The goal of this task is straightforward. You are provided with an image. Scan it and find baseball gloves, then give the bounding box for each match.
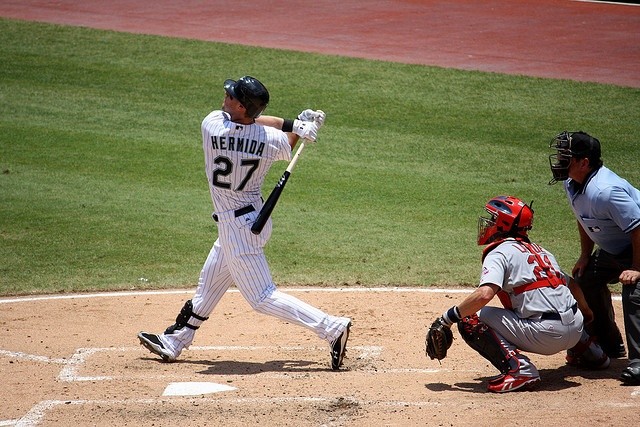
[425,318,454,361]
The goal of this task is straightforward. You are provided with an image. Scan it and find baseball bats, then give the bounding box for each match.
[250,110,326,236]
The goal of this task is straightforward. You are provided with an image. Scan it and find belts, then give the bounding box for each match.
[212,197,264,222]
[542,303,578,320]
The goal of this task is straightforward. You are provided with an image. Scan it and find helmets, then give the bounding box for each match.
[548,131,601,185]
[477,195,535,245]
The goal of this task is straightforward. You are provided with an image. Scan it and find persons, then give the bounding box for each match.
[543,132,640,386]
[138,75,352,371]
[425,194,612,393]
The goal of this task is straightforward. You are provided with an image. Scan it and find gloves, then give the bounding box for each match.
[292,119,317,142]
[298,108,326,126]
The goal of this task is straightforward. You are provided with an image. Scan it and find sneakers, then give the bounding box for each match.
[618,361,640,383]
[488,373,541,393]
[138,331,174,362]
[330,316,353,370]
[565,347,610,370]
[599,340,626,357]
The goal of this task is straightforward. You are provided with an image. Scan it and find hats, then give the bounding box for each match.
[223,76,269,118]
